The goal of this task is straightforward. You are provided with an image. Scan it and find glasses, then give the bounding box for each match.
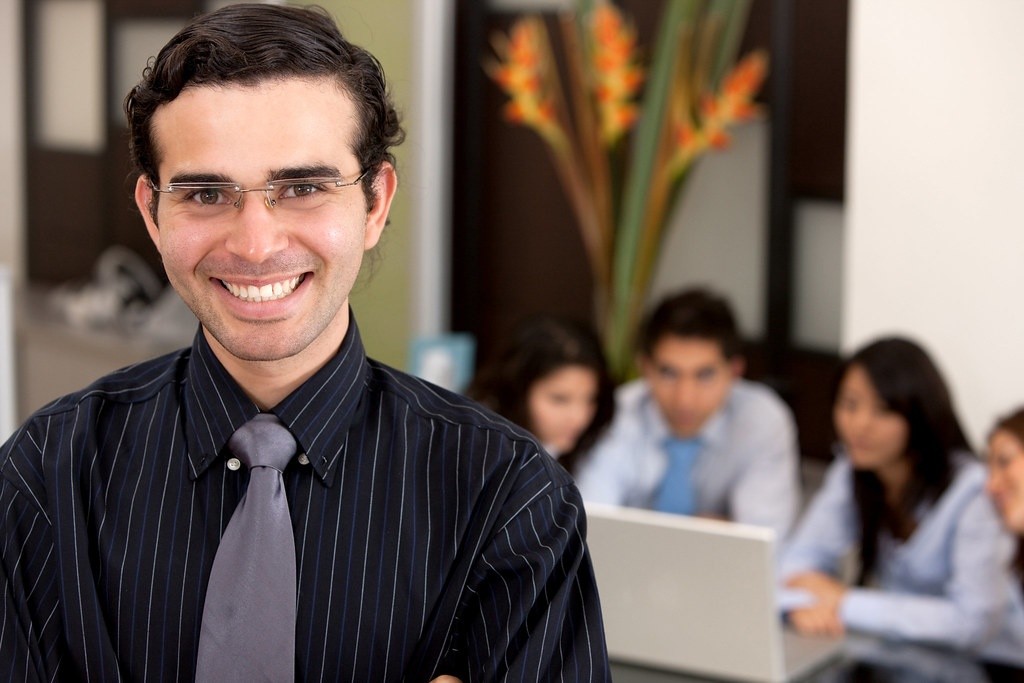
[146,167,373,223]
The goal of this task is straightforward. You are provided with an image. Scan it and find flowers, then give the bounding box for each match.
[483,0,778,390]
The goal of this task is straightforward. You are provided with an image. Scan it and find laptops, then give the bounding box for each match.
[580,504,849,683]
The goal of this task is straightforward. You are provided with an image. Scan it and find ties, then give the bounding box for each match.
[649,441,702,515]
[195,415,296,683]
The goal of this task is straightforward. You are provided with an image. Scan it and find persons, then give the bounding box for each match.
[571,290,797,556]
[0,5,611,683]
[782,341,1024,682]
[463,311,616,476]
